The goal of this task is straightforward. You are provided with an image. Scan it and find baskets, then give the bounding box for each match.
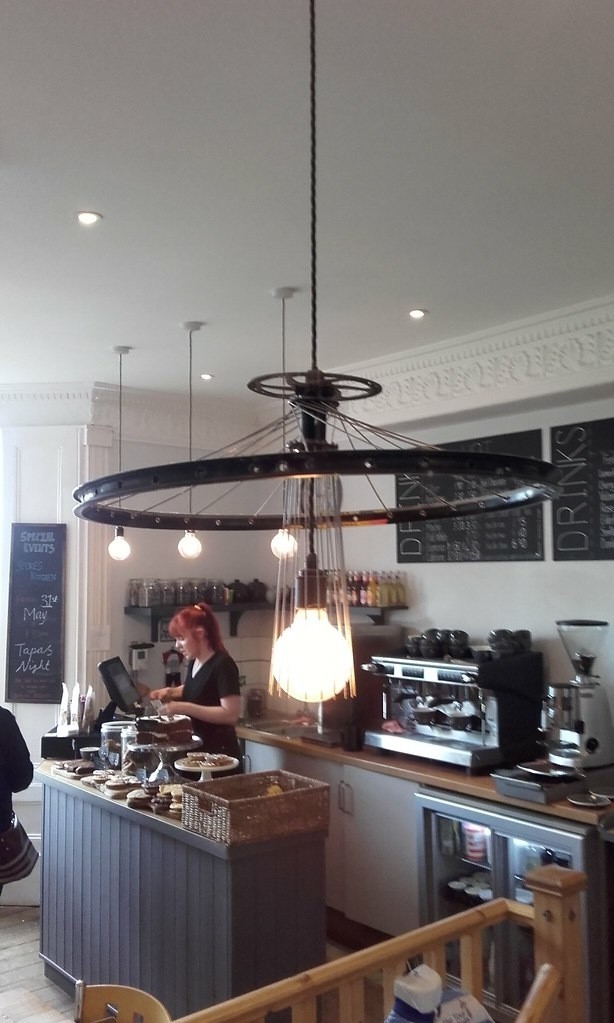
[181,769,331,844]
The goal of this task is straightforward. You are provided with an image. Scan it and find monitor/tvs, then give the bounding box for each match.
[98,656,141,712]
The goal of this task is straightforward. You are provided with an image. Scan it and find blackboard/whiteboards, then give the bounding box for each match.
[395,429,545,564]
[549,417,614,561]
[5,523,68,704]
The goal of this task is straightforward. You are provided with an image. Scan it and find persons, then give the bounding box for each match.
[148,602,244,781]
[0,707,33,896]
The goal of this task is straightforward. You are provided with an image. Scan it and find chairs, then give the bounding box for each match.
[75,979,171,1023]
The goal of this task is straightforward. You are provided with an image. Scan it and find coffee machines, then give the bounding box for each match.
[536,620,614,770]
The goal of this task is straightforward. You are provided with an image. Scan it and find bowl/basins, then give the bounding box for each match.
[80,747,100,761]
[448,870,535,905]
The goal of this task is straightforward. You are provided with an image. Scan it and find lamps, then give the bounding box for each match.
[71,1,567,705]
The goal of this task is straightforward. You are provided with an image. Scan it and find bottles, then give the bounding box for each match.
[326,569,405,608]
[128,577,290,605]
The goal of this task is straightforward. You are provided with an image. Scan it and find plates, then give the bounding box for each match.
[566,787,614,806]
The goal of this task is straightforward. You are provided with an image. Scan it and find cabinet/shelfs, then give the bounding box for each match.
[234,739,422,941]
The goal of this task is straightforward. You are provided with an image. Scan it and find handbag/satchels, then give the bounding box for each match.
[0,814,39,885]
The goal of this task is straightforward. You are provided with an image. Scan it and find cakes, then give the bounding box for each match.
[135,713,193,746]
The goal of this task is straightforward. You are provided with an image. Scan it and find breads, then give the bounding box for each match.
[63,761,190,812]
[186,752,228,766]
